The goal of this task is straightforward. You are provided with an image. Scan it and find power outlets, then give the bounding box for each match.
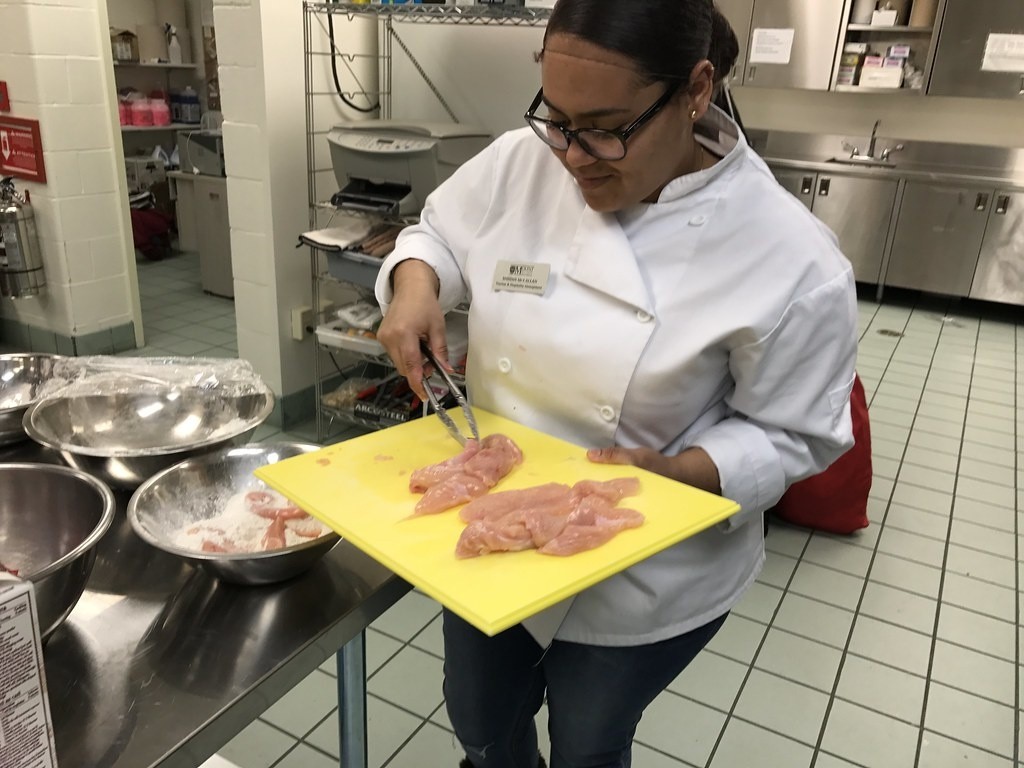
[312,299,334,335]
[292,305,316,341]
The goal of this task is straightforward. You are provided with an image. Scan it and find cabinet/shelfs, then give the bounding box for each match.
[193,180,234,299]
[112,59,208,254]
[767,166,901,301]
[301,0,556,443]
[876,181,1024,306]
[830,0,947,96]
[927,0,1024,100]
[713,0,846,91]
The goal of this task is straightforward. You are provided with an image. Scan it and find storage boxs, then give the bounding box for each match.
[175,130,225,176]
[123,155,166,193]
[109,25,146,61]
[0,567,59,768]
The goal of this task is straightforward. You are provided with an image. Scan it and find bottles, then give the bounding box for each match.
[151,145,170,171]
[117,91,171,126]
[170,145,179,171]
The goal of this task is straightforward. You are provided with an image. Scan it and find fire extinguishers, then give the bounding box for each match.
[0,177,46,297]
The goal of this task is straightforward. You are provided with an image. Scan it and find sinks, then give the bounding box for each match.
[825,156,897,169]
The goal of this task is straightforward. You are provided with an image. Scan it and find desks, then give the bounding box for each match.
[0,349,415,768]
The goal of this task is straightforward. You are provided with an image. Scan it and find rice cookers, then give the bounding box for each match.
[169,88,201,123]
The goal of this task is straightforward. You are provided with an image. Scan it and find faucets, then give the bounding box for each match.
[867,120,881,157]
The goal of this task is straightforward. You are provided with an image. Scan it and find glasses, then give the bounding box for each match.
[524,81,684,161]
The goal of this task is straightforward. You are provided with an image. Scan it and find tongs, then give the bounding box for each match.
[418,338,480,448]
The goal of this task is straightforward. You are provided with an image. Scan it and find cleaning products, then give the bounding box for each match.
[164,21,182,63]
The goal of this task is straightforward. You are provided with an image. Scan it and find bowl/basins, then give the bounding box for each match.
[129,443,335,590]
[0,462,117,640]
[0,353,87,458]
[22,356,276,496]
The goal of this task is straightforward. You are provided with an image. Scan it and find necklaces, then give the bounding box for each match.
[697,144,706,172]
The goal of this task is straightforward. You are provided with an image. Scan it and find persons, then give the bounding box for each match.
[374,0,858,768]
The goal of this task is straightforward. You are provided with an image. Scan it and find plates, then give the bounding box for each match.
[255,406,743,637]
